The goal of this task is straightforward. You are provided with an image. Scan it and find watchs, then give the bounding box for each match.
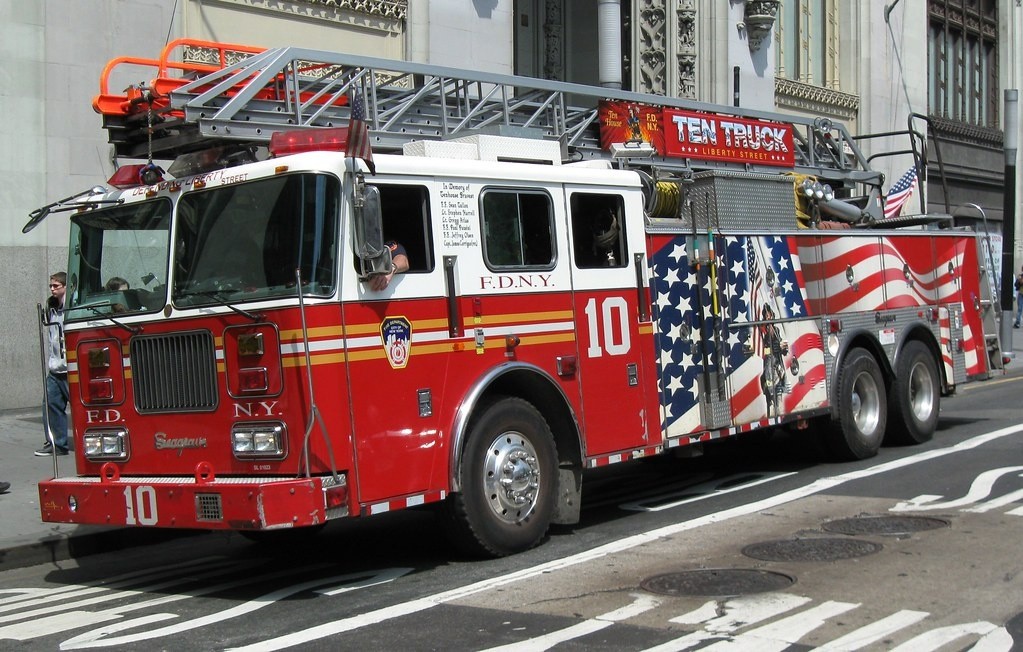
[391,263,398,273]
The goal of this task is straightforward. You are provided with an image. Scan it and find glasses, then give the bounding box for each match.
[70,285,74,289]
[49,284,63,288]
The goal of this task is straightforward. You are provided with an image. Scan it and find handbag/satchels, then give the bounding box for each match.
[1015,279,1021,290]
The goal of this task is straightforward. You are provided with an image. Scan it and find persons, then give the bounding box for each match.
[368,237,409,292]
[1011,265,1023,329]
[105,278,129,292]
[761,303,792,394]
[33,272,77,457]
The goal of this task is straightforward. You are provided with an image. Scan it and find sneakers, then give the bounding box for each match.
[34,441,68,455]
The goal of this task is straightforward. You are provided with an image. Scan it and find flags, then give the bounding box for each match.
[883,159,921,219]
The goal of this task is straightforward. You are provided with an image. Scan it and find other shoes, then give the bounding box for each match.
[1014,321,1020,328]
[0,481,11,493]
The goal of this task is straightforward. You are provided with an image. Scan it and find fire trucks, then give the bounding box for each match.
[22,38,1011,561]
